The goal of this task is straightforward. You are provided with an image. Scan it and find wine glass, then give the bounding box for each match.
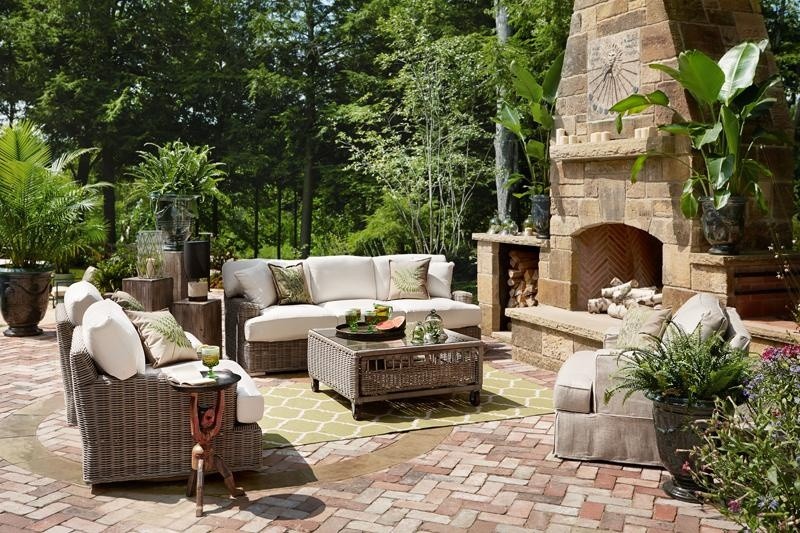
[345,308,378,334]
[202,346,220,380]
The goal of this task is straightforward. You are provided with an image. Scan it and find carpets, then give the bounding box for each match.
[251,365,555,449]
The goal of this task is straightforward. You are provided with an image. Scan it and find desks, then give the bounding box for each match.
[122,252,189,316]
[167,370,245,517]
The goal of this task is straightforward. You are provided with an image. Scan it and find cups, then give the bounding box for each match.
[524,228,532,236]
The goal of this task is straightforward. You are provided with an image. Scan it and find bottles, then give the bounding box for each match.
[411,309,443,344]
[487,214,534,236]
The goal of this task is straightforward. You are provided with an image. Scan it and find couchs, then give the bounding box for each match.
[54,281,264,484]
[222,254,482,377]
[553,293,752,467]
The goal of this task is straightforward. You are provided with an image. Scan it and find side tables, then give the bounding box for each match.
[173,298,222,359]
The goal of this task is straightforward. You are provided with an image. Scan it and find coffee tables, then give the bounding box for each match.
[308,322,485,420]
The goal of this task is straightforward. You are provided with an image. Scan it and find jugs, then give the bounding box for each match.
[374,304,393,325]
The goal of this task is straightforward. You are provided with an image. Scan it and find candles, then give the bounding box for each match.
[146,258,156,278]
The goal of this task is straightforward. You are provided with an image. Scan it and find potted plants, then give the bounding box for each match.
[123,137,233,251]
[608,37,796,256]
[604,317,763,506]
[489,49,566,239]
[0,117,116,337]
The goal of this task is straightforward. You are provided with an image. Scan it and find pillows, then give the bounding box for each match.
[124,307,201,369]
[388,257,432,300]
[617,302,671,353]
[426,261,455,298]
[234,261,277,310]
[112,291,144,311]
[266,262,315,305]
